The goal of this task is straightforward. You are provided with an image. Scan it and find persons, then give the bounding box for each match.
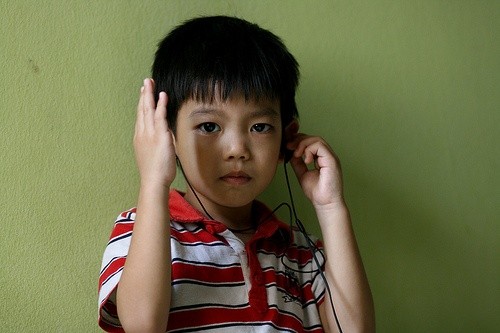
[97,16,376,333]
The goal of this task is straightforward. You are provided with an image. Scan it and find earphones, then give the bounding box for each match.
[281,139,295,163]
